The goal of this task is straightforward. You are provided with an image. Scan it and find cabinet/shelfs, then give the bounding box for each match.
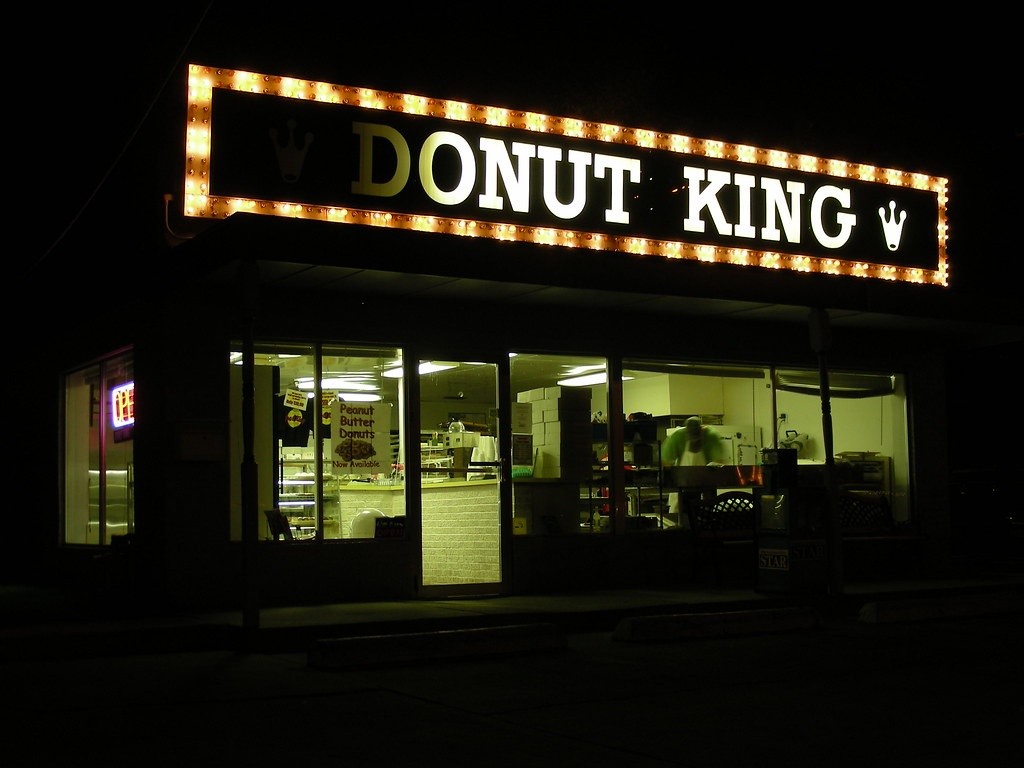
[279,447,341,539]
[388,417,496,480]
[585,411,665,533]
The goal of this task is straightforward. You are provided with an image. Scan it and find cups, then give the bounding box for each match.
[467,435,498,480]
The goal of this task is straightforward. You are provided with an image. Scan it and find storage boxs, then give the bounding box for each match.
[495,385,594,536]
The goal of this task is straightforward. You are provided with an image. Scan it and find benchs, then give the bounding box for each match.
[684,489,929,590]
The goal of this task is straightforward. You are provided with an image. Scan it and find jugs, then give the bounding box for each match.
[449,417,465,433]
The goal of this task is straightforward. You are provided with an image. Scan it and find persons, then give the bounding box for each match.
[657,415,728,514]
